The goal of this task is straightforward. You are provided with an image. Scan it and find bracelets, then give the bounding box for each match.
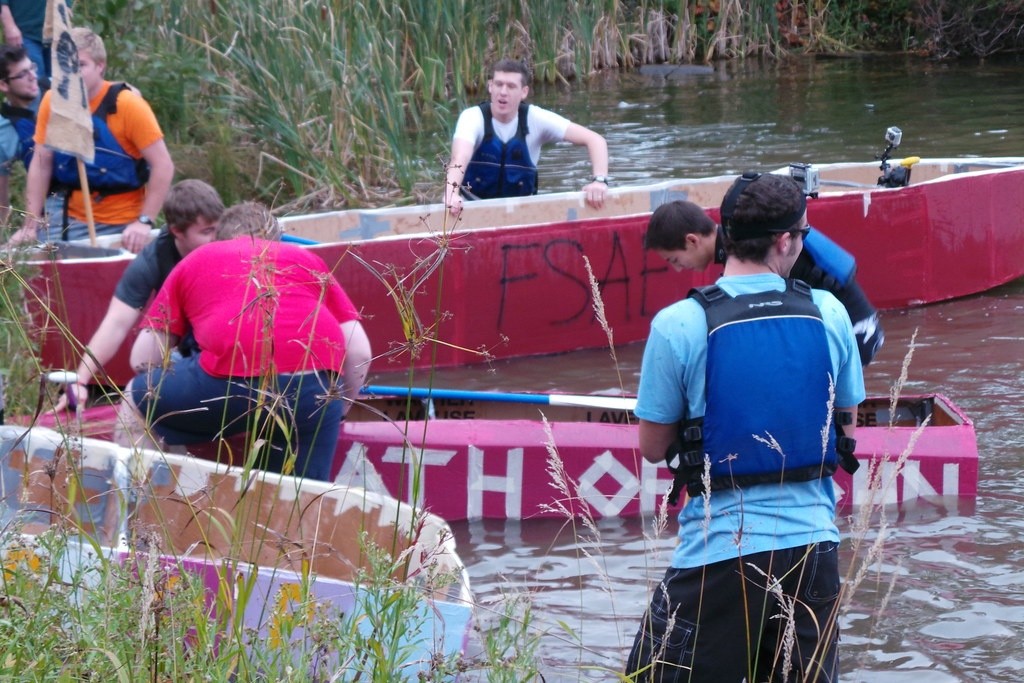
[341,415,347,421]
[76,381,87,387]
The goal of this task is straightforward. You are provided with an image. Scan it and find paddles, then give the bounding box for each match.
[360,384,637,411]
[280,234,320,245]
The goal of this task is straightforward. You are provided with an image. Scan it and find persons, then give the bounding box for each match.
[0,0,372,483]
[645,201,885,369]
[625,172,867,683]
[443,57,608,216]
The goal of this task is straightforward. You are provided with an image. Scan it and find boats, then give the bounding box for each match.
[1,392,977,522]
[0,156,1023,388]
[1,425,478,683]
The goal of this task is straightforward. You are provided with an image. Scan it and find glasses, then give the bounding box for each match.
[768,224,810,240]
[4,62,37,80]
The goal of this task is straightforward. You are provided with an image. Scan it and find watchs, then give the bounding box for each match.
[137,215,156,229]
[592,175,607,186]
